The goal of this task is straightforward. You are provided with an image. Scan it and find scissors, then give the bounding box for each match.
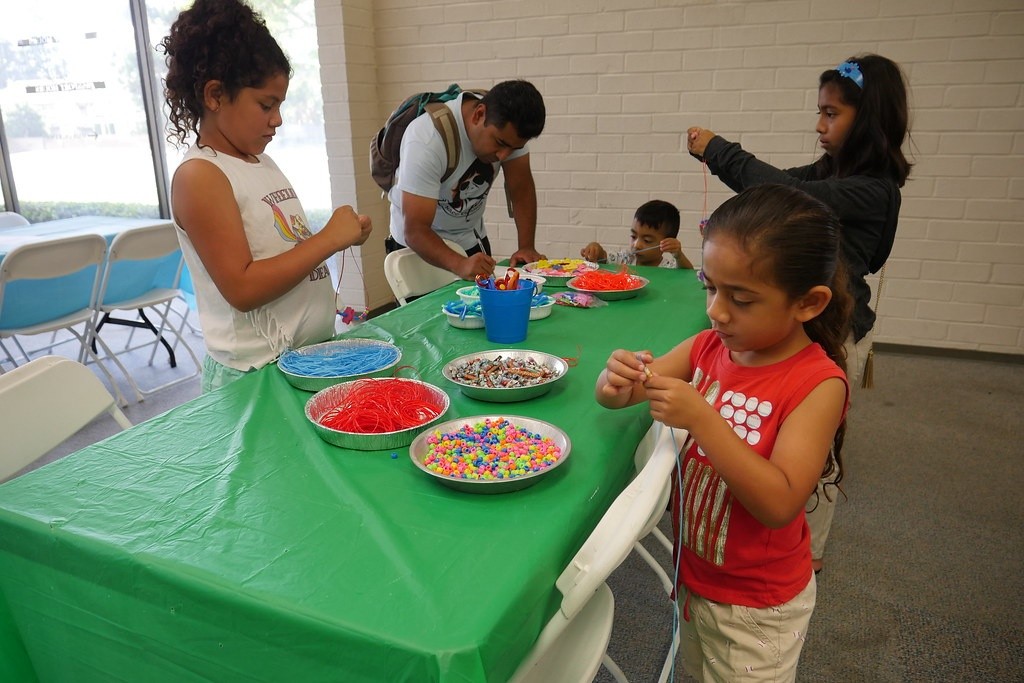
[507,272,519,290]
[476,274,489,287]
[494,268,516,290]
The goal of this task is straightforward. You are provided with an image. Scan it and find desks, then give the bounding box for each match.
[0,214,179,369]
[0,252,715,683]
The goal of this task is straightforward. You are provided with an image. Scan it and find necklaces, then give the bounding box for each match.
[697,152,710,283]
[334,243,374,325]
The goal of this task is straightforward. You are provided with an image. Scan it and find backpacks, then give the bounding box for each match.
[369,84,490,192]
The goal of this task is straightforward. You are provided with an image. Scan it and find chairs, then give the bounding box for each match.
[83,222,202,404]
[0,233,128,411]
[384,237,470,309]
[508,415,691,683]
[0,211,30,232]
[0,354,136,490]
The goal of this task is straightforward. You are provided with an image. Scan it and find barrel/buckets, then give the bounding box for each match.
[475,278,538,344]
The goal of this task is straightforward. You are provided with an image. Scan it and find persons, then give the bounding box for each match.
[156,0,374,391]
[580,199,694,269]
[369,79,553,309]
[594,184,851,683]
[687,52,916,574]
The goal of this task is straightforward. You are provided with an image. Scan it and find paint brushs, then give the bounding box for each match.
[473,228,497,279]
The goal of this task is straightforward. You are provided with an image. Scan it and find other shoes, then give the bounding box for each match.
[808,559,822,575]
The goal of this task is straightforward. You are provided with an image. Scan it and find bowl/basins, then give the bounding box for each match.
[442,308,485,329]
[522,260,600,287]
[528,294,557,321]
[303,377,451,451]
[408,414,572,494]
[456,286,481,305]
[566,273,650,301]
[443,348,569,403]
[277,338,403,393]
[518,275,546,294]
